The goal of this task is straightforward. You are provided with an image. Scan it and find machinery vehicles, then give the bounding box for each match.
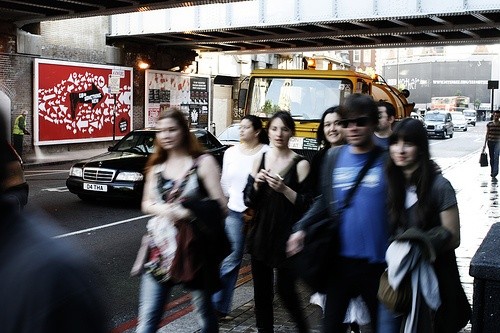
[231,57,416,154]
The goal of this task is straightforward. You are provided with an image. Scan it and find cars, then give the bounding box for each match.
[452,111,467,131]
[218,122,245,148]
[424,111,453,139]
[66,129,228,201]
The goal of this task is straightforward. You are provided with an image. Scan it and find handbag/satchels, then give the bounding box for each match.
[130,235,152,276]
[294,215,342,295]
[479,148,488,167]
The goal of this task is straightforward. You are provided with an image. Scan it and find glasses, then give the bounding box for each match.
[338,117,372,128]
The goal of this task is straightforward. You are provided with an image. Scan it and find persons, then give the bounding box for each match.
[220,92,471,333]
[129,107,234,333]
[12,110,30,160]
[479,110,500,182]
[1,90,113,333]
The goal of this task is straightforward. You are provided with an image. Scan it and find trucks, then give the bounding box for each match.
[462,107,476,127]
